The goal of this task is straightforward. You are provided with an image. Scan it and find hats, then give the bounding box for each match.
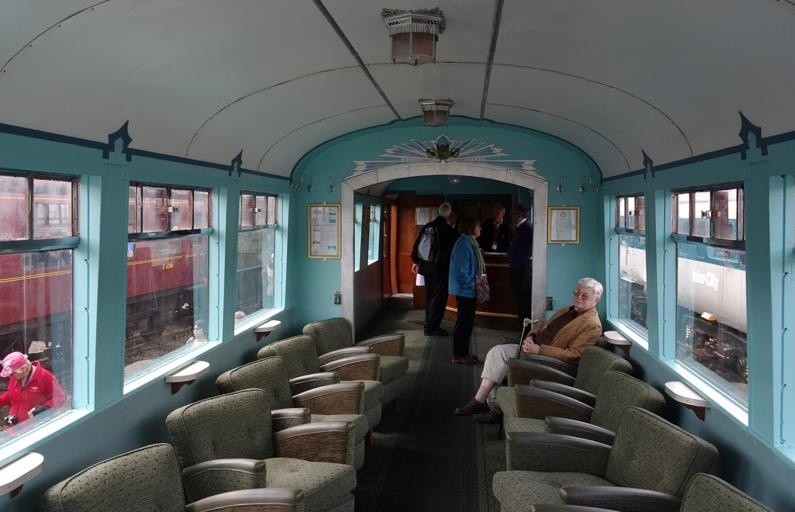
[0,352,29,378]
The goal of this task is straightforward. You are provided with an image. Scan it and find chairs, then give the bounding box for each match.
[302,317,410,412]
[216,355,369,470]
[486,334,606,412]
[530,472,771,512]
[41,443,304,512]
[496,344,633,416]
[492,406,720,512]
[166,388,357,512]
[503,371,666,445]
[256,335,383,443]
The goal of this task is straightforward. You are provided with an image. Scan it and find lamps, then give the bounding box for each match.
[449,176,462,184]
[417,97,453,127]
[382,7,445,66]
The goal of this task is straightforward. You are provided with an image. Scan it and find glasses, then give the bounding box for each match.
[573,288,589,297]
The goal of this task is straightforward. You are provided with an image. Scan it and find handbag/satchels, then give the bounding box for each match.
[476,273,491,304]
[417,233,435,262]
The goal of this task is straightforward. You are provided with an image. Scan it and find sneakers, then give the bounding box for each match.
[455,397,490,415]
[475,408,503,422]
[453,354,478,365]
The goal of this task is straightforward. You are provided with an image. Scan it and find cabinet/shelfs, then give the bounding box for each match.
[448,193,512,235]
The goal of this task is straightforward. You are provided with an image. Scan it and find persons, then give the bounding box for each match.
[0,351,67,432]
[448,215,488,365]
[235,311,246,320]
[454,278,603,425]
[473,201,516,257]
[186,319,208,343]
[507,202,533,333]
[411,202,459,336]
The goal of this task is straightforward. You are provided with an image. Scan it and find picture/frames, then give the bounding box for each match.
[308,203,342,259]
[548,206,580,244]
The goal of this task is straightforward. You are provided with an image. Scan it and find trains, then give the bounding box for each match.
[617,189,747,364]
[1,176,269,390]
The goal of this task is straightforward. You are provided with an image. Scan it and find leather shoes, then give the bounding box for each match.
[424,328,449,336]
